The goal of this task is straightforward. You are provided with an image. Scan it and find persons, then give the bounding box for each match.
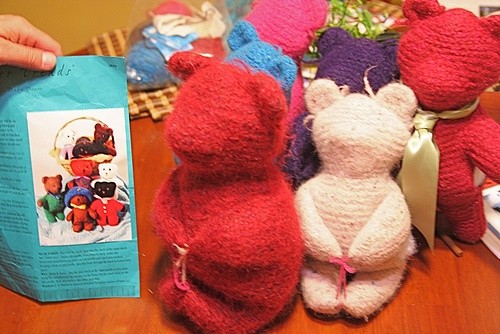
[0,14,64,74]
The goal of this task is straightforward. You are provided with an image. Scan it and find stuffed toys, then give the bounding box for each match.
[64,185,96,232]
[59,127,76,159]
[148,51,303,333]
[240,0,330,59]
[126,40,169,91]
[89,182,127,225]
[295,77,418,320]
[284,27,397,191]
[218,20,298,91]
[92,123,117,156]
[72,137,92,158]
[37,174,64,223]
[64,160,95,188]
[90,163,125,190]
[397,1,500,243]
